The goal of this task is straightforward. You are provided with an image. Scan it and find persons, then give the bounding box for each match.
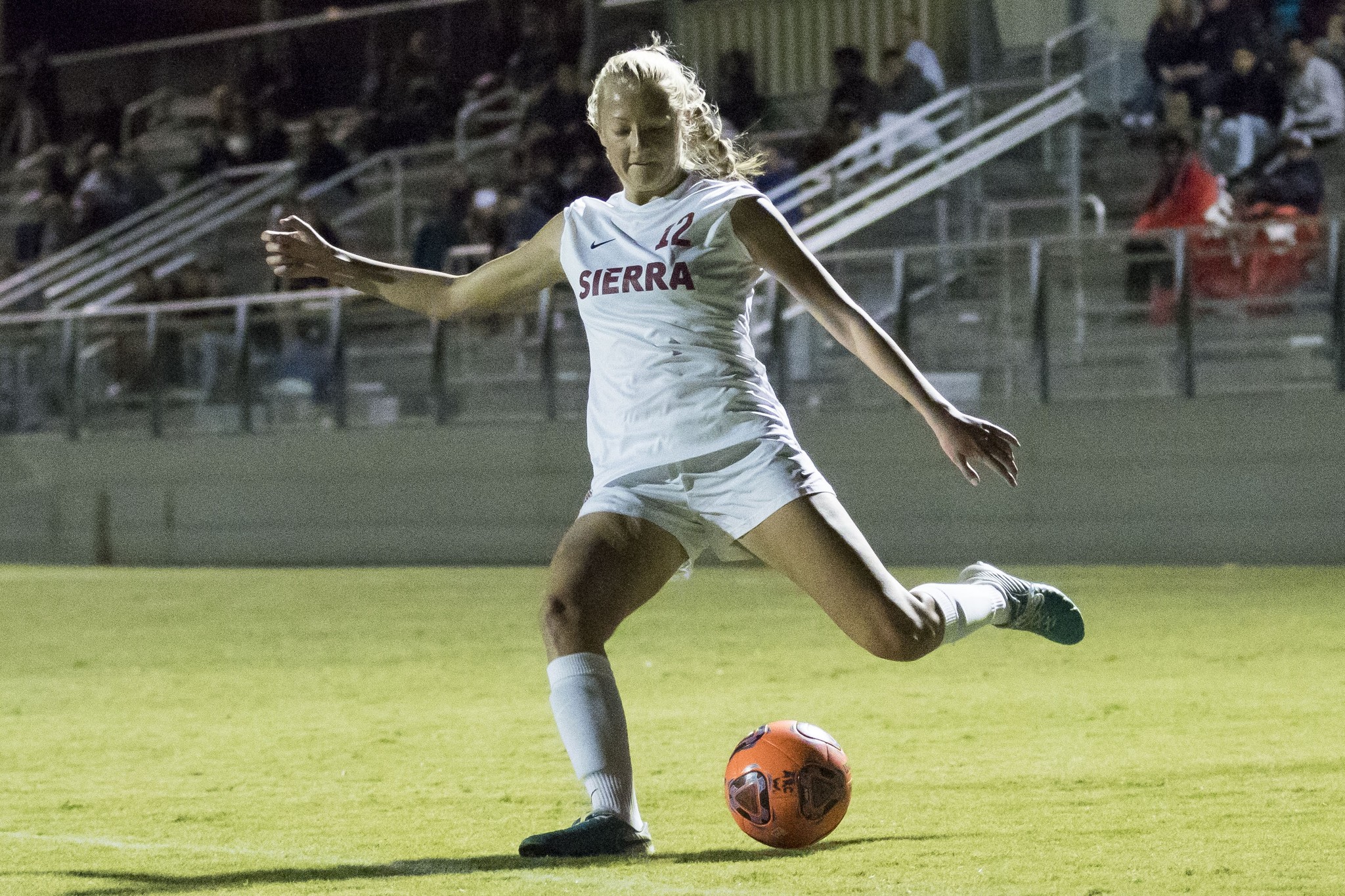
[0,1,952,400]
[1112,0,1340,319]
[256,46,1089,860]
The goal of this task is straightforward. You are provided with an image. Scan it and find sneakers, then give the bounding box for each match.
[959,560,1086,646]
[518,806,655,858]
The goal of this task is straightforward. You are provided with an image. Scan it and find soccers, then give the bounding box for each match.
[727,720,857,850]
